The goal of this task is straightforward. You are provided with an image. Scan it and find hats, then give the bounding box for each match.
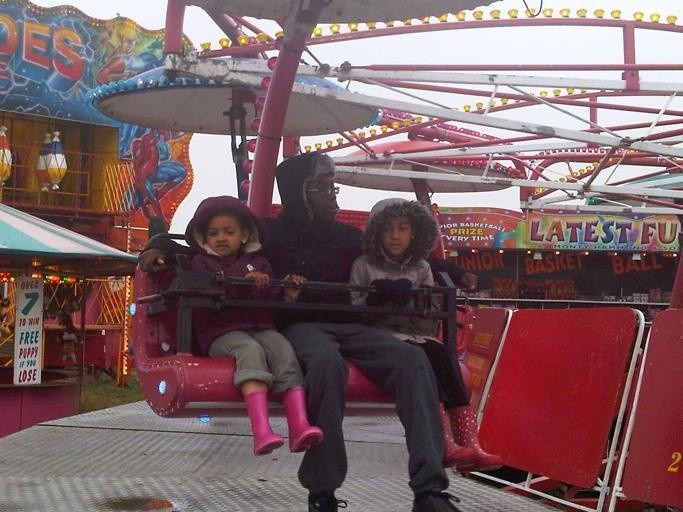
[272,152,335,221]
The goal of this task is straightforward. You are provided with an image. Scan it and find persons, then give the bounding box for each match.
[136,150,479,512]
[187,195,325,455]
[348,199,505,475]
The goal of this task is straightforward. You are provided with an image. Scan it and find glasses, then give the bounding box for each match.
[307,186,340,196]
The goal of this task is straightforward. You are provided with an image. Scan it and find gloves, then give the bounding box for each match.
[365,275,416,309]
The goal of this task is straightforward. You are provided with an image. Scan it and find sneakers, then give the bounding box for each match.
[411,490,463,512]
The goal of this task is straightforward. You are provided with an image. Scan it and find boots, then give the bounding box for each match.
[439,403,475,469]
[449,403,505,475]
[245,390,284,457]
[282,384,325,453]
